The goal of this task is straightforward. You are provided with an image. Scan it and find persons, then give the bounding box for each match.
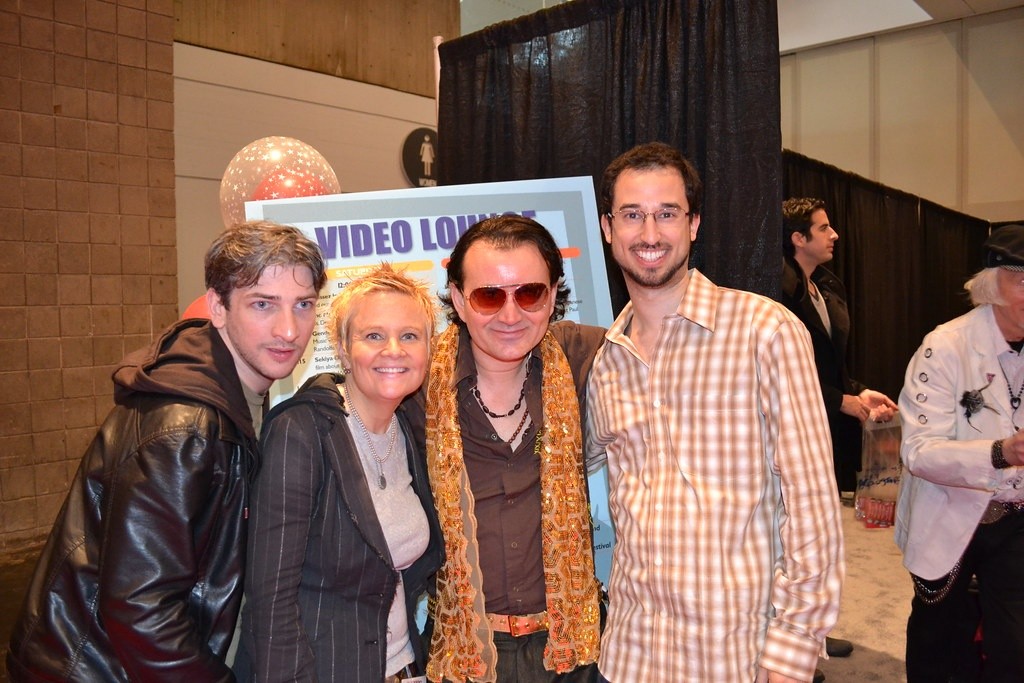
[583,144,845,683]
[6,222,328,683]
[415,215,609,683]
[228,261,447,682]
[895,226,1024,683]
[781,198,899,492]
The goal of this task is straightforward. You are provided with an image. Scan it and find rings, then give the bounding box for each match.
[882,419,885,423]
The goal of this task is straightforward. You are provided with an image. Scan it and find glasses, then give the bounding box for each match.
[605,207,693,228]
[454,281,553,316]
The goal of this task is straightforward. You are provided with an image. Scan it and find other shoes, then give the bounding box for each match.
[825,635,854,657]
[813,668,826,682]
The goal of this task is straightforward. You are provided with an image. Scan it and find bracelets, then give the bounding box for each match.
[991,440,1013,469]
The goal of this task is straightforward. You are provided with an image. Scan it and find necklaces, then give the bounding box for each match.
[997,356,1024,409]
[344,383,395,489]
[473,353,529,445]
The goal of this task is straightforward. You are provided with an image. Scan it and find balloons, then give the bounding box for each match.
[220,136,341,230]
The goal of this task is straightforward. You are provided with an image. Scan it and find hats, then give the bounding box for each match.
[982,224,1024,271]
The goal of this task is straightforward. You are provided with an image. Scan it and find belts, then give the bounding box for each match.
[474,607,550,639]
